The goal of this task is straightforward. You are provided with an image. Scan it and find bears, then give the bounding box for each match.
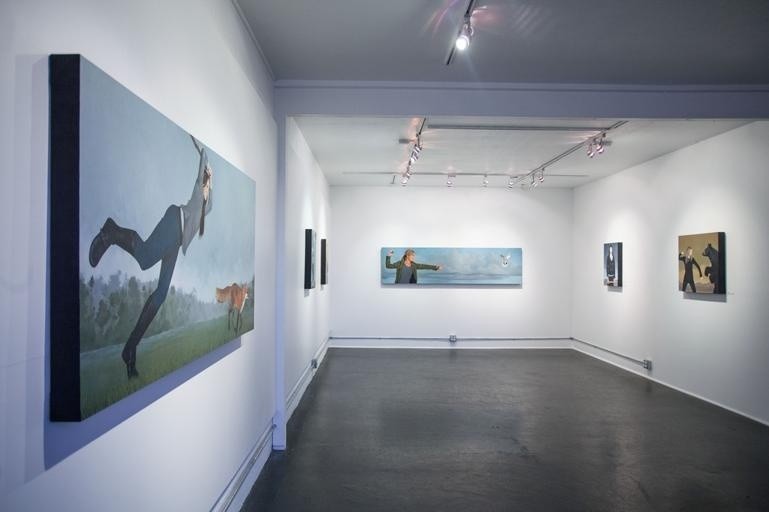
[702,243,720,293]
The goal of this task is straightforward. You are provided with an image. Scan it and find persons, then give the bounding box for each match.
[89,148,215,376]
[386,250,442,284]
[679,247,703,293]
[606,246,617,286]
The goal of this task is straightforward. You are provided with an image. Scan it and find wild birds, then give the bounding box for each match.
[500,254,511,265]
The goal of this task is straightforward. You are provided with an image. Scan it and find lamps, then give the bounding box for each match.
[586,136,605,160]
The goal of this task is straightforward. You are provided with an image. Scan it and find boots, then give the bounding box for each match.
[89,218,137,268]
[122,295,158,379]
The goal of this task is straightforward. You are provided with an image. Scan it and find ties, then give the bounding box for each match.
[199,198,207,235]
